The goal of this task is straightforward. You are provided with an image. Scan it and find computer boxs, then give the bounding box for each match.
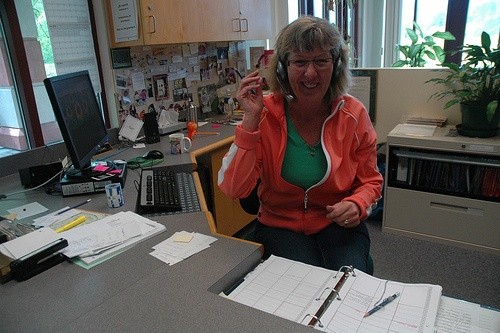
[61,159,127,196]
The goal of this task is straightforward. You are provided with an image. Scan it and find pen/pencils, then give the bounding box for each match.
[233,66,268,113]
[362,291,400,319]
[54,198,91,218]
[195,130,221,135]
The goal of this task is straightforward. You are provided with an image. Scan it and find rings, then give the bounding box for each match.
[344,218,349,225]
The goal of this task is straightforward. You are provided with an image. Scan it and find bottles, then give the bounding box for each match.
[224,88,232,112]
[186,105,198,127]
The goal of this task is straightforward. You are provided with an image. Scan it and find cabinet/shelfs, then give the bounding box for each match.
[382,124,500,255]
[102,0,247,48]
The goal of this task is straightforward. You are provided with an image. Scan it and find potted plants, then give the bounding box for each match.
[424,31,500,138]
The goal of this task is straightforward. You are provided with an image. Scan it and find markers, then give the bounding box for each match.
[47,216,86,232]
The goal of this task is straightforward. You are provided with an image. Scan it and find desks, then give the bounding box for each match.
[0,118,500,333]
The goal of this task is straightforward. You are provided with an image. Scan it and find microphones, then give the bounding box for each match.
[277,77,294,101]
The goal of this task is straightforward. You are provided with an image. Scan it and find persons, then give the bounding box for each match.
[218,15,384,275]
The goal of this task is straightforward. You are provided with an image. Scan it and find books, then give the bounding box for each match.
[408,156,500,199]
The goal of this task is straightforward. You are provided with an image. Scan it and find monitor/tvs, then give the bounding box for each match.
[43,69,115,179]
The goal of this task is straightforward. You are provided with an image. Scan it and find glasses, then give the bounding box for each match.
[283,54,335,71]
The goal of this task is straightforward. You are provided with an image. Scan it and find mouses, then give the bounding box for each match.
[142,150,164,160]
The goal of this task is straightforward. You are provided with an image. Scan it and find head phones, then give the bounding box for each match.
[276,45,343,81]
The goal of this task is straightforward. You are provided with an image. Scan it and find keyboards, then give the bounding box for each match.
[140,170,183,211]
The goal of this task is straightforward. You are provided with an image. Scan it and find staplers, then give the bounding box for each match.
[9,237,68,282]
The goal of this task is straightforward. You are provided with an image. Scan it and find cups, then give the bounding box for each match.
[187,122,197,136]
[169,133,191,155]
